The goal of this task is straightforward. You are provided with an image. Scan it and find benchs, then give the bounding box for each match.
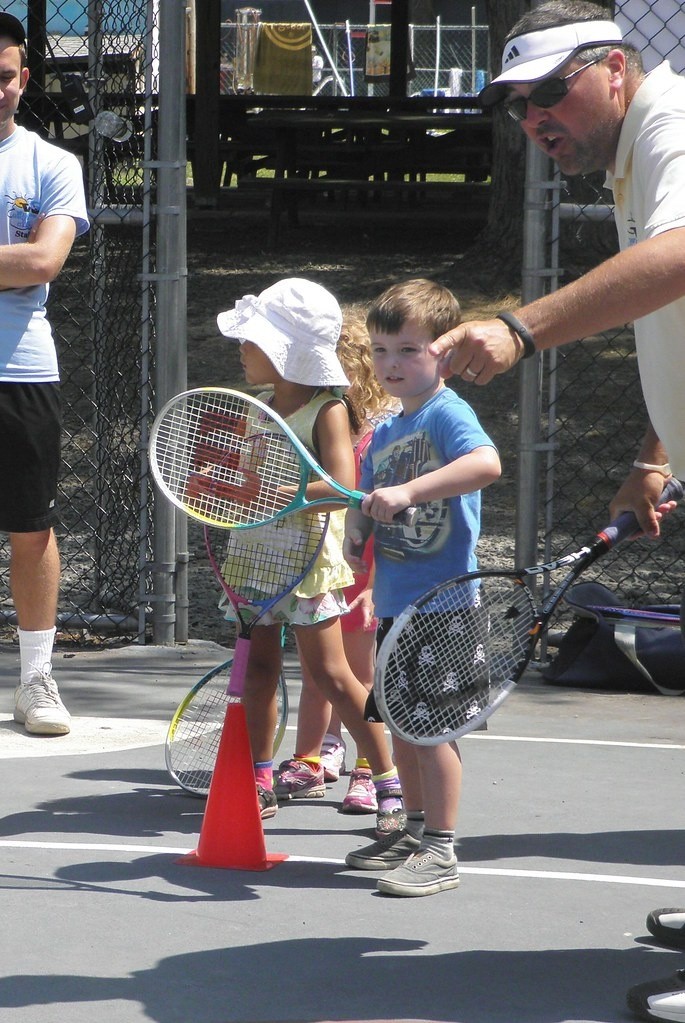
[89,94,487,232]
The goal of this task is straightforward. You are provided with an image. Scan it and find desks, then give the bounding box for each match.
[250,104,489,220]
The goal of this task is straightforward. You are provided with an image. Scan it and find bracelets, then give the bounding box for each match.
[632,459,672,476]
[495,312,535,359]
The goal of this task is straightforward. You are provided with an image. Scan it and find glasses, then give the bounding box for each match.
[222,56,228,59]
[503,55,609,122]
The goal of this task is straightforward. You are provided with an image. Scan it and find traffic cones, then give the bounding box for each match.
[170,703,290,874]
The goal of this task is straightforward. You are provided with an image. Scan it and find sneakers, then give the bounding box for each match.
[646,908,685,949]
[345,829,421,870]
[374,788,407,839]
[272,759,326,800]
[343,767,378,813]
[321,733,346,781]
[377,849,460,896]
[256,782,278,818]
[13,661,71,734]
[627,973,685,1023]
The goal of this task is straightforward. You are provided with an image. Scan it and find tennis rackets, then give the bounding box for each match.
[148,387,420,530]
[205,511,331,696]
[373,478,685,747]
[165,622,289,799]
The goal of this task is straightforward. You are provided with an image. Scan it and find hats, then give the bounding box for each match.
[0,12,27,54]
[217,278,351,398]
[477,19,624,106]
[311,46,317,51]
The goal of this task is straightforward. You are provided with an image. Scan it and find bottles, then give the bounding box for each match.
[95,111,132,142]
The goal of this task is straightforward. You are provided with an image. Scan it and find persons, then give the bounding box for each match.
[271,300,383,812]
[217,277,407,838]
[321,705,347,781]
[0,11,90,732]
[427,0,685,1023]
[342,277,500,896]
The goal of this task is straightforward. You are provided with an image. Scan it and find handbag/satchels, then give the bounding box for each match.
[543,581,685,696]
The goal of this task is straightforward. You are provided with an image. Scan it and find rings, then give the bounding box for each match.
[466,366,478,377]
